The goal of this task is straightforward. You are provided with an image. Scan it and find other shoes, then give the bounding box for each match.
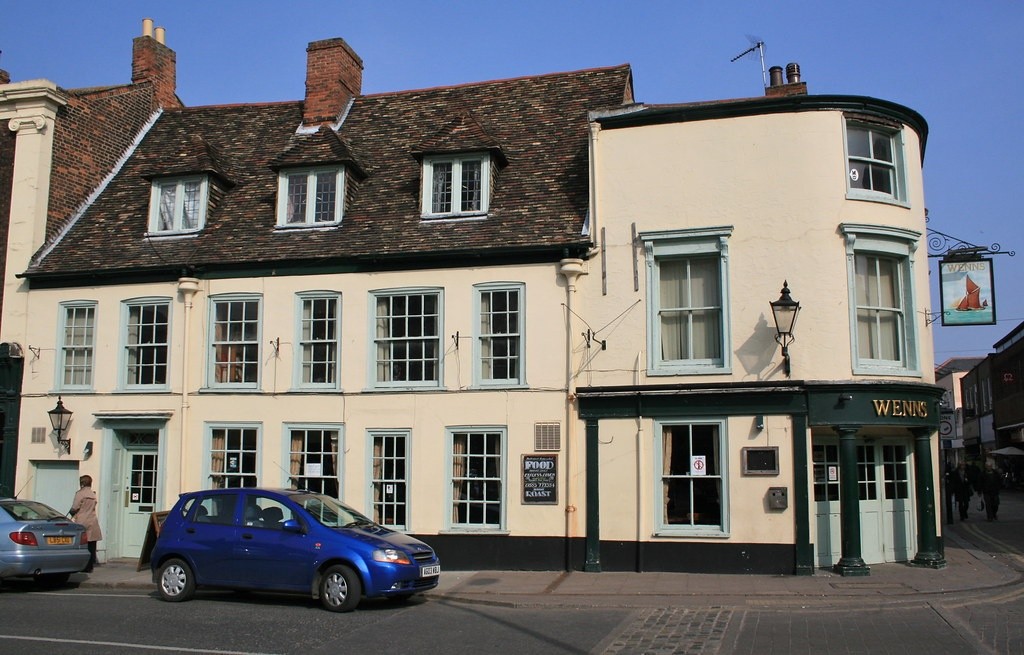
[81,567,93,572]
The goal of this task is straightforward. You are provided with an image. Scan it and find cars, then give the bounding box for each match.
[0,497,92,588]
[149,488,441,612]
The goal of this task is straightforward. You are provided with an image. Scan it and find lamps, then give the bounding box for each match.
[769,279,802,376]
[47,395,73,456]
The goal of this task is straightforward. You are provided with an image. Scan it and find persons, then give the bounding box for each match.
[946,459,1003,522]
[69,475,102,573]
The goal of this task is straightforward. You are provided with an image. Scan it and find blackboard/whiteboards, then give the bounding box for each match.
[137,511,171,572]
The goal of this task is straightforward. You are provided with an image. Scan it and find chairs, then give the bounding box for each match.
[247,505,283,530]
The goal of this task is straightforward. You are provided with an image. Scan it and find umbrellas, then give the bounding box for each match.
[988,446,1024,463]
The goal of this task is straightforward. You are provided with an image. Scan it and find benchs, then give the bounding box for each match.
[196,506,213,522]
